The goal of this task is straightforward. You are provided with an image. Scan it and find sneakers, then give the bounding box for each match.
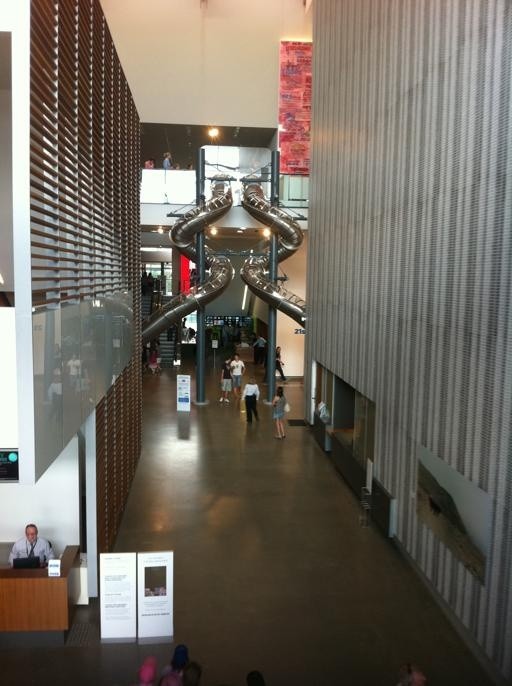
[220,397,229,402]
[274,433,286,439]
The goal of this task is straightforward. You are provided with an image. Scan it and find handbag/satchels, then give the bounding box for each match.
[283,403,290,413]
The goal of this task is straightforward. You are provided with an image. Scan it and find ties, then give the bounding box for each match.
[28,542,35,556]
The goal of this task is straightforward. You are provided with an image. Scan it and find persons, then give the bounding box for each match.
[272,386,287,439]
[158,644,189,686]
[8,525,54,567]
[47,333,120,423]
[246,671,265,686]
[397,662,425,685]
[139,656,157,686]
[144,151,195,171]
[142,269,290,426]
[183,661,201,685]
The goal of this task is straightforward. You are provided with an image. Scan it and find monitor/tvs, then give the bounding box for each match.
[13,556,40,569]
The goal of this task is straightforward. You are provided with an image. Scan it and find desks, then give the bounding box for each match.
[0,545,82,632]
